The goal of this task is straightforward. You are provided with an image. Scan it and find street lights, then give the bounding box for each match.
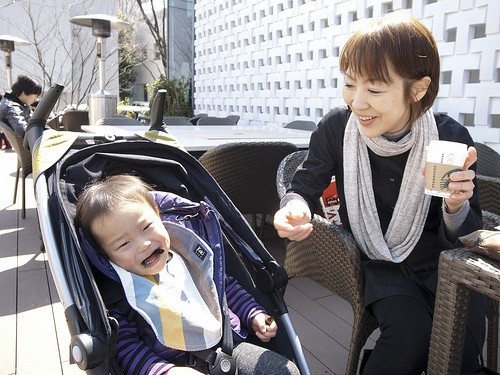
[69,13,131,124]
[0,34,31,89]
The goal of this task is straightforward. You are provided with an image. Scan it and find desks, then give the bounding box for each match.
[427,242,500,374]
[81,122,313,156]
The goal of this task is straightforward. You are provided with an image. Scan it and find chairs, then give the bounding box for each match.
[1,103,500,374]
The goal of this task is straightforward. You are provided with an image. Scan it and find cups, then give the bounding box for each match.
[424,140,468,198]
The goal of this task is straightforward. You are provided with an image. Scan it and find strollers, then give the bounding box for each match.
[22,83,311,375]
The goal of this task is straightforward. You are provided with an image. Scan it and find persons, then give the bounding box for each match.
[0,74,42,137]
[77,174,301,375]
[273,17,482,375]
